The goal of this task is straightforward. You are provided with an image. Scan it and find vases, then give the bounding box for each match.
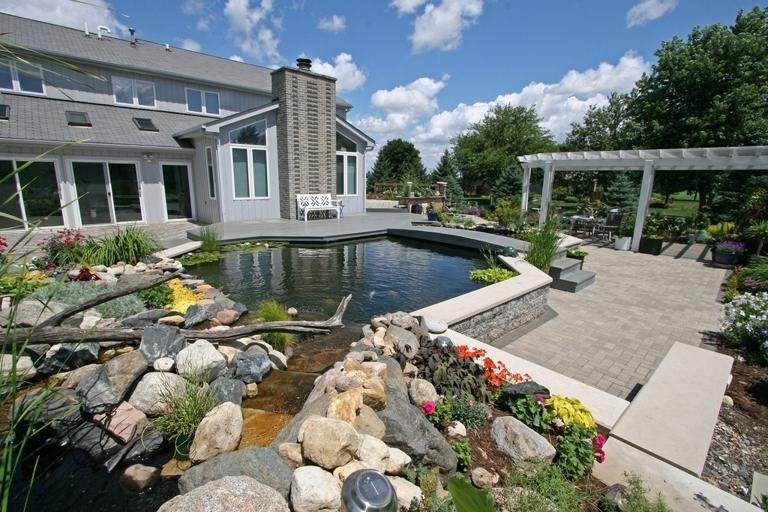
[710,248,743,265]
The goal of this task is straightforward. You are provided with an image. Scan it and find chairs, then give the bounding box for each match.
[592,213,618,240]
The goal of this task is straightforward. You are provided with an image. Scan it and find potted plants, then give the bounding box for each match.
[566,248,589,270]
[638,215,665,256]
[657,215,711,244]
[614,234,632,250]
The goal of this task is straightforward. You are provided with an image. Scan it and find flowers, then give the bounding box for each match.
[714,240,747,254]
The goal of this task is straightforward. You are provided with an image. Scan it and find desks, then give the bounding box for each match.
[571,215,594,238]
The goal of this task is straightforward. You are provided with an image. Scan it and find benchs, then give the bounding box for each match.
[295,193,340,222]
